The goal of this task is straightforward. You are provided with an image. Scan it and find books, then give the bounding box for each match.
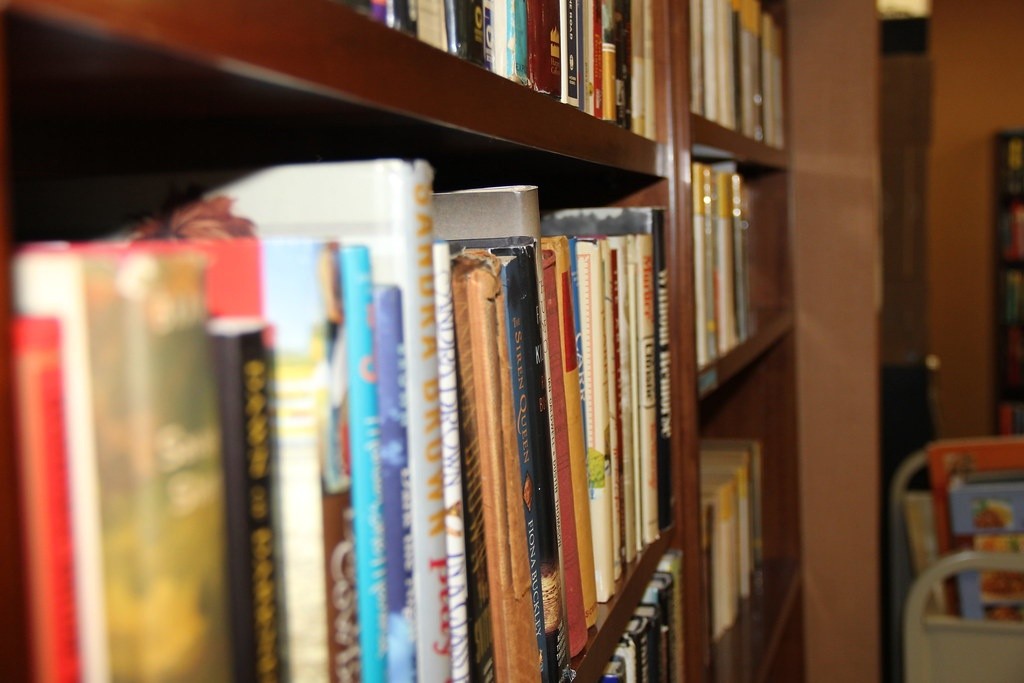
[691,0,785,148]
[0,156,683,683]
[604,439,764,683]
[694,160,757,372]
[360,1,657,139]
[903,437,1024,623]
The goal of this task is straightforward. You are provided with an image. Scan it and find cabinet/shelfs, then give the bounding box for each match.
[0,0,806,683]
[991,126,1024,438]
